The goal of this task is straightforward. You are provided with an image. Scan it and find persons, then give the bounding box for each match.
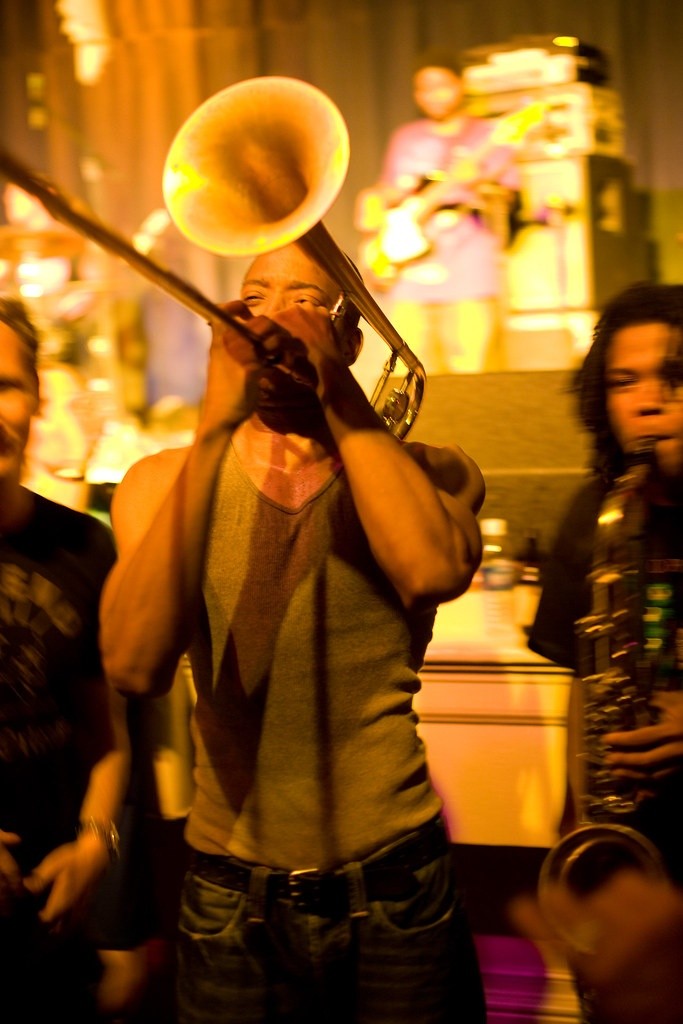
[525,280,682,1024]
[0,296,196,1024]
[359,60,527,377]
[97,243,488,1024]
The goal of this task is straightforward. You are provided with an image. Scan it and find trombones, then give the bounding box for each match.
[0,72,426,448]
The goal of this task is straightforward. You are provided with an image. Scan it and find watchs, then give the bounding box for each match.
[76,819,119,862]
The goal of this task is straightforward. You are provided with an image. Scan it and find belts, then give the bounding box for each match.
[194,817,450,918]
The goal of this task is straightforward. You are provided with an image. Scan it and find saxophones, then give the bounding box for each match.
[534,434,683,968]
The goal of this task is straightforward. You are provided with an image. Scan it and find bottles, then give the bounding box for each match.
[469,518,517,590]
[515,528,550,585]
